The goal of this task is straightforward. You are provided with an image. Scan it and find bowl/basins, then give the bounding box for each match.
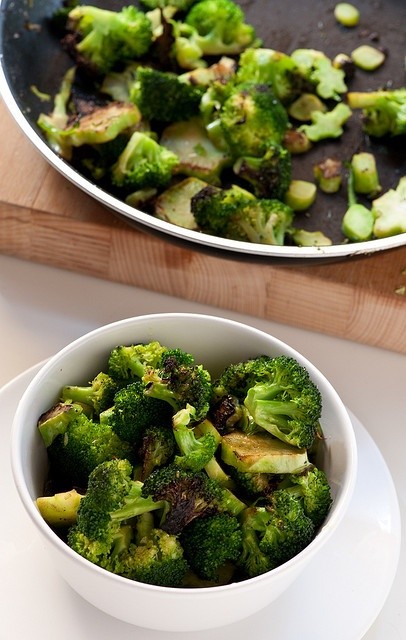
[1,1,406,262]
[8,310,359,636]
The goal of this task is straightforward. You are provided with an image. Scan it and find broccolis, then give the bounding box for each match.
[36,343,332,585]
[39,1,406,244]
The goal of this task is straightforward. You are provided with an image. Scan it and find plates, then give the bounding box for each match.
[0,352,403,640]
